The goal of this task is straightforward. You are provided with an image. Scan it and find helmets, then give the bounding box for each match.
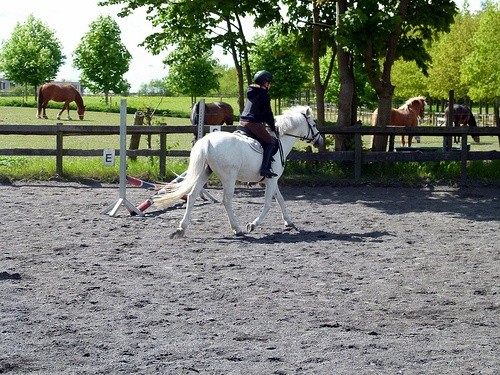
[254,70,272,85]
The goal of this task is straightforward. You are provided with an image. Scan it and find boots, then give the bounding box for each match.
[260,147,277,177]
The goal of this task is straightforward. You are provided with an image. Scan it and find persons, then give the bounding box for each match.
[237,69,278,178]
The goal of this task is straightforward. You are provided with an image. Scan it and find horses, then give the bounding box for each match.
[373,97,425,146]
[155,105,323,239]
[191,102,233,144]
[37,84,85,121]
[445,104,480,143]
